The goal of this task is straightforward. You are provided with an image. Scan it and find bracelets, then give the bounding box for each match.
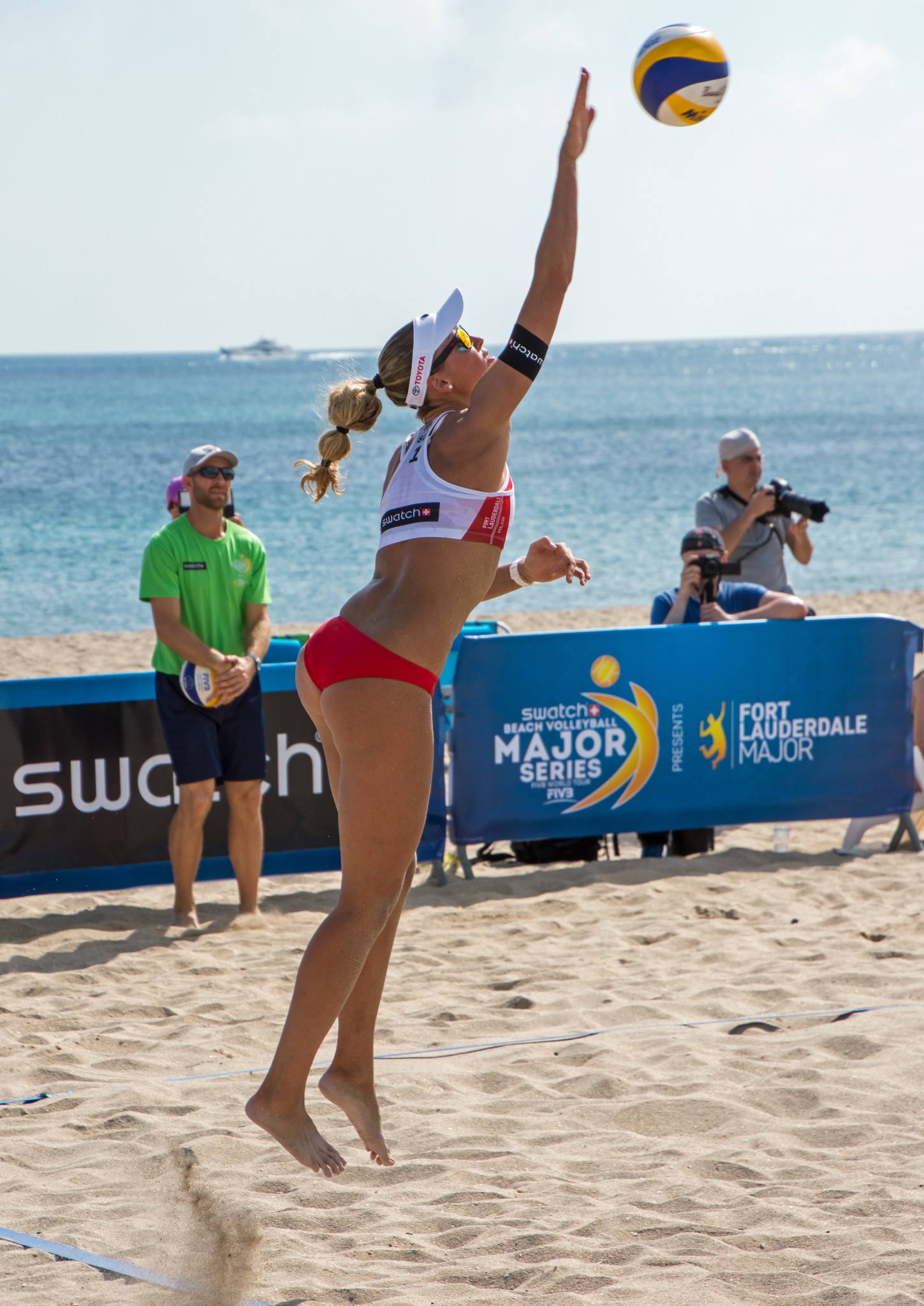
[494,321,549,382]
[508,556,533,587]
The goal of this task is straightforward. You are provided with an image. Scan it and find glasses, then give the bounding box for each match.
[740,454,762,462]
[191,465,235,481]
[431,324,473,373]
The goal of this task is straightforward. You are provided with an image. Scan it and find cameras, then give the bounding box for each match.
[685,554,743,580]
[763,478,832,524]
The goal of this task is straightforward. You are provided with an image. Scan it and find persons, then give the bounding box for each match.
[139,445,275,931]
[694,426,813,618]
[635,526,809,859]
[243,67,596,1181]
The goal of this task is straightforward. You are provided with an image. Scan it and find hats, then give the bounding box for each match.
[406,288,464,411]
[183,444,238,474]
[679,526,726,552]
[716,428,761,477]
[166,476,186,503]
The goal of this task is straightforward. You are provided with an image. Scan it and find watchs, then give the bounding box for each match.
[247,654,262,671]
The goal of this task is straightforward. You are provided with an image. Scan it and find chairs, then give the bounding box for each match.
[435,617,516,860]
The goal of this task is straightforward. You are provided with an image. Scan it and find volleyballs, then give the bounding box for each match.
[633,22,730,127]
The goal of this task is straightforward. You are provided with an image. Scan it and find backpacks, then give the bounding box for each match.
[473,834,603,862]
